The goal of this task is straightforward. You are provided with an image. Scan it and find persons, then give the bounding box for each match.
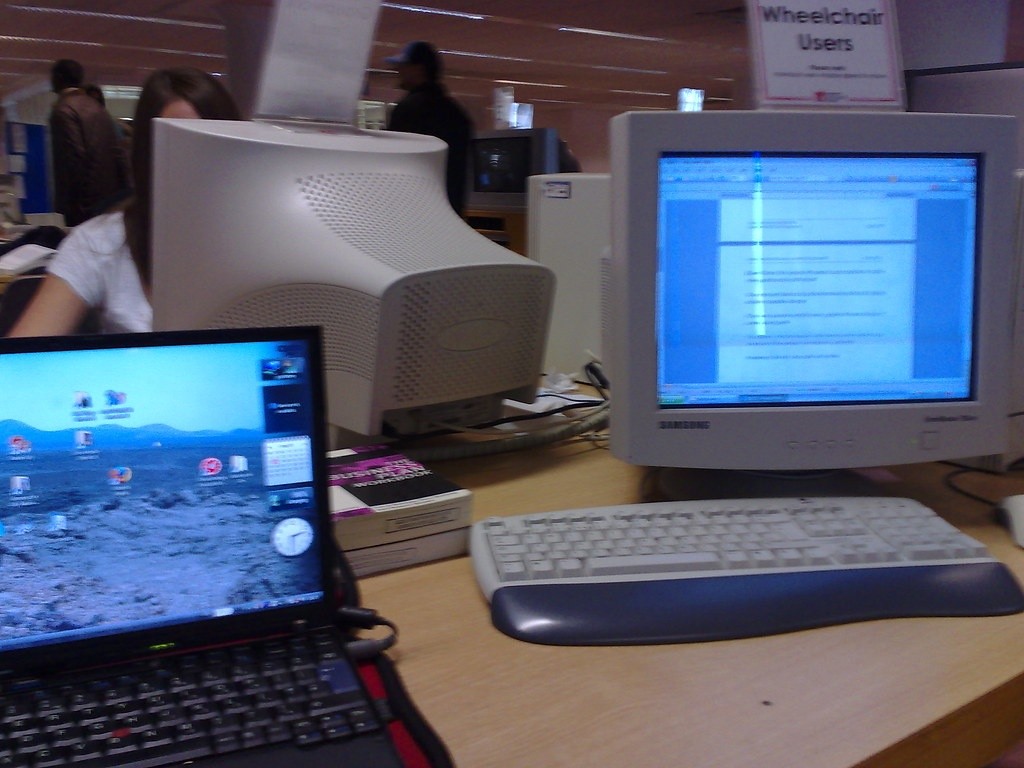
[49,58,132,227]
[384,41,474,218]
[5,68,247,338]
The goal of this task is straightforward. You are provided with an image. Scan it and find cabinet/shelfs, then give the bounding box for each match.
[465,211,527,256]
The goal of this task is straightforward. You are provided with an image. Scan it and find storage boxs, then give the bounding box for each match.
[323,443,472,577]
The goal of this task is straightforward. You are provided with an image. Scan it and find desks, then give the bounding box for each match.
[359,384,1024,767]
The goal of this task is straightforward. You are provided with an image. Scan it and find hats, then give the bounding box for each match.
[385,42,442,74]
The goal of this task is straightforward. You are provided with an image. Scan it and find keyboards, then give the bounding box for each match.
[467,493,1024,647]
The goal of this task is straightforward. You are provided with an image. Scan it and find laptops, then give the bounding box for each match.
[0,323,415,768]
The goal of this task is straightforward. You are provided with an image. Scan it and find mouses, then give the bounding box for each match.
[997,494,1024,549]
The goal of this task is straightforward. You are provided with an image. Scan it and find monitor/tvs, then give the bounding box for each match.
[146,116,558,451]
[601,104,1018,501]
[520,173,609,388]
[460,126,571,212]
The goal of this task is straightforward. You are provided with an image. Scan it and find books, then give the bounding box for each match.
[319,442,472,579]
